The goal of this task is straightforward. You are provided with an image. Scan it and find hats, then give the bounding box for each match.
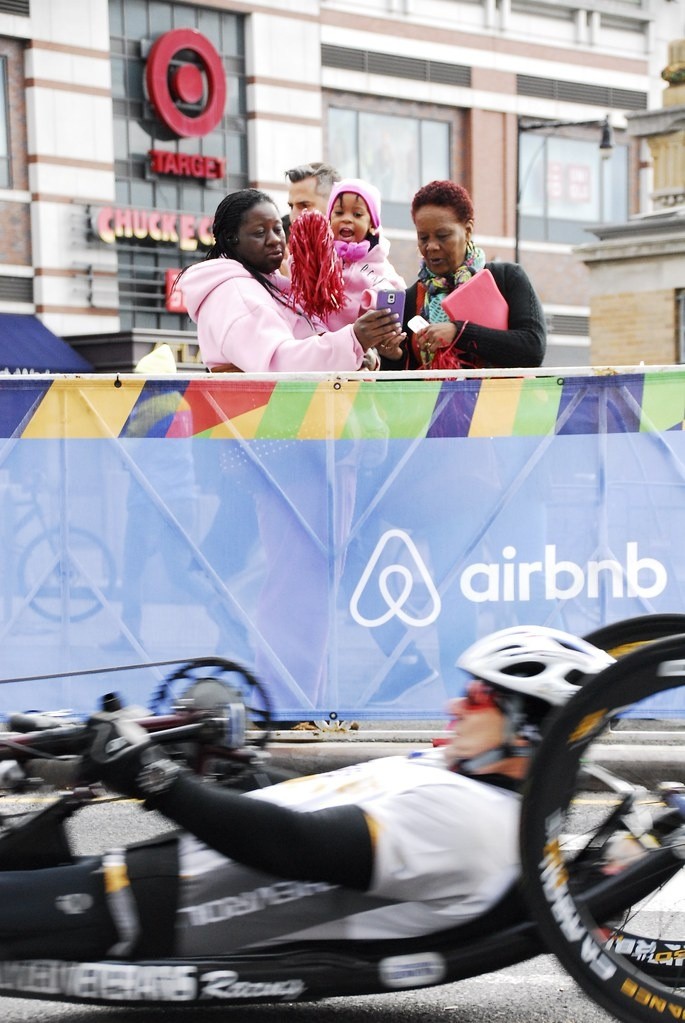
[326,178,381,229]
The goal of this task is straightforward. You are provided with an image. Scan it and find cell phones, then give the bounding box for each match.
[378,289,406,335]
[409,314,430,334]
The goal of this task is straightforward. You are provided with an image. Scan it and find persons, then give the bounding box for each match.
[171,160,547,732]
[0,625,617,963]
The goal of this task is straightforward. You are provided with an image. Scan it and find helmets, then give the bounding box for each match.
[457,626,641,748]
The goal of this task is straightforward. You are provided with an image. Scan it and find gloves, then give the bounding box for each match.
[85,713,190,800]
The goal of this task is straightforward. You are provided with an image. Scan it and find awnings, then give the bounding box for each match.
[0,314,94,375]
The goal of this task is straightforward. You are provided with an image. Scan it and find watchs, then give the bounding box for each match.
[138,757,179,812]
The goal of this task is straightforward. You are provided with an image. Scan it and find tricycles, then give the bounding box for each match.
[0,613,685,1022]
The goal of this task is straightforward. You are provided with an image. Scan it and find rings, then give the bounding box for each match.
[381,344,385,347]
[386,346,392,349]
[427,342,431,347]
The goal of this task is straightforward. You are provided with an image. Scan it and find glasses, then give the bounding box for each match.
[460,682,502,712]
